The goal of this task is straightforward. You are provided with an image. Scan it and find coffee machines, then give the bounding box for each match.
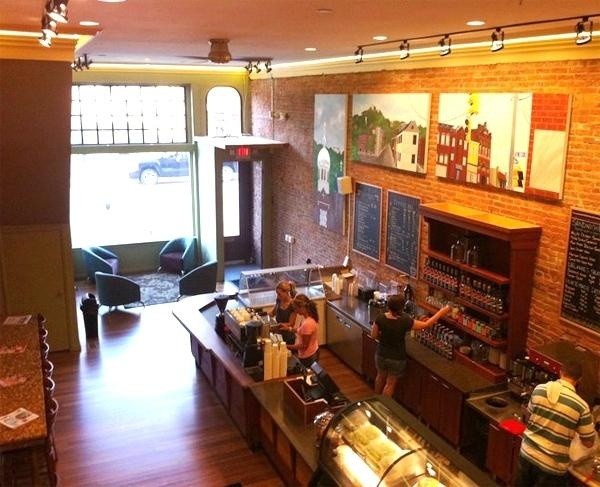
[506,338,600,409]
[213,292,230,334]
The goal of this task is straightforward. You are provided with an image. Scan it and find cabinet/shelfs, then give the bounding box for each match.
[484,420,523,487]
[394,353,420,416]
[239,263,327,349]
[412,196,546,382]
[420,363,508,451]
[362,326,379,387]
[314,393,498,487]
[326,301,363,376]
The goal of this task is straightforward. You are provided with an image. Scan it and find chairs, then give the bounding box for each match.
[32,311,63,482]
[177,259,218,302]
[83,245,118,280]
[95,270,143,313]
[154,234,199,275]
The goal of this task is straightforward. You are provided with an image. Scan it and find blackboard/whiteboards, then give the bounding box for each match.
[558,206,600,338]
[351,180,382,263]
[384,189,422,281]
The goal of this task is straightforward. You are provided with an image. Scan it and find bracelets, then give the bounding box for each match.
[289,326,292,331]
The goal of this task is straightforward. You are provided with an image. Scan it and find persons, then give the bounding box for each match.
[367,293,450,397]
[513,358,595,486]
[267,280,299,354]
[280,294,320,368]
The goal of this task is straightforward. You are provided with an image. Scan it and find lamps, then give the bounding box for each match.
[353,10,597,70]
[72,50,96,76]
[244,60,273,77]
[38,0,70,50]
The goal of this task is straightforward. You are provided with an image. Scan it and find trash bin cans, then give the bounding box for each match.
[80,292,99,338]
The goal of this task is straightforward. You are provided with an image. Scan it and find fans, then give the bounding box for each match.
[176,38,259,65]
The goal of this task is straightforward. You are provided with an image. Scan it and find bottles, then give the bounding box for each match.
[413,256,504,360]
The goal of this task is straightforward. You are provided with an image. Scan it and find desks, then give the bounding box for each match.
[0,310,51,487]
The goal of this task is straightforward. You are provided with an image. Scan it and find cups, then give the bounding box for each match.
[257,336,289,381]
[331,262,402,306]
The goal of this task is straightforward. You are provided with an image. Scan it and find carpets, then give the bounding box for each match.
[121,272,184,308]
